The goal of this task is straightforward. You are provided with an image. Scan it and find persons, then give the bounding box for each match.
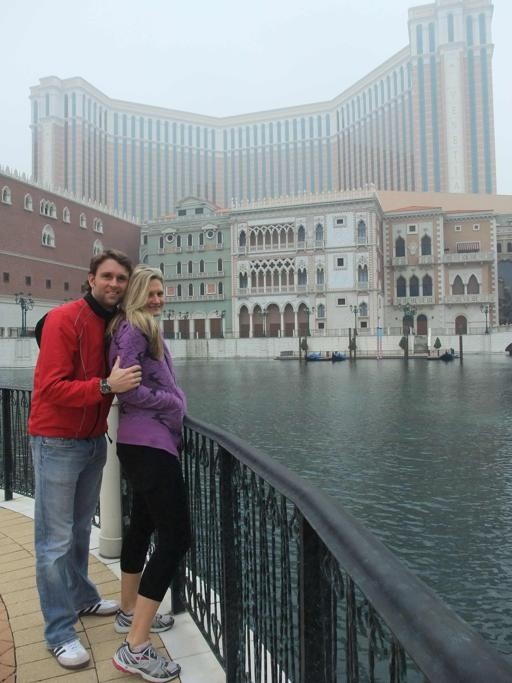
[108,258,192,683]
[27,249,144,671]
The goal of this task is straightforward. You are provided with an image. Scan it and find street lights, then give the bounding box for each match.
[258,308,270,338]
[403,302,417,334]
[15,292,35,337]
[216,310,226,337]
[303,307,314,336]
[179,312,189,339]
[479,303,494,333]
[349,304,362,336]
[163,309,175,339]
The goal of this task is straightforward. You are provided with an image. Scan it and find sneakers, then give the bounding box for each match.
[51,639,91,669]
[114,609,176,635]
[78,599,120,616]
[113,639,181,682]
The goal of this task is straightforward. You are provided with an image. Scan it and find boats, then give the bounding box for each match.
[441,350,454,362]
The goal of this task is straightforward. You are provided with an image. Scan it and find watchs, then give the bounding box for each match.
[100,378,112,395]
